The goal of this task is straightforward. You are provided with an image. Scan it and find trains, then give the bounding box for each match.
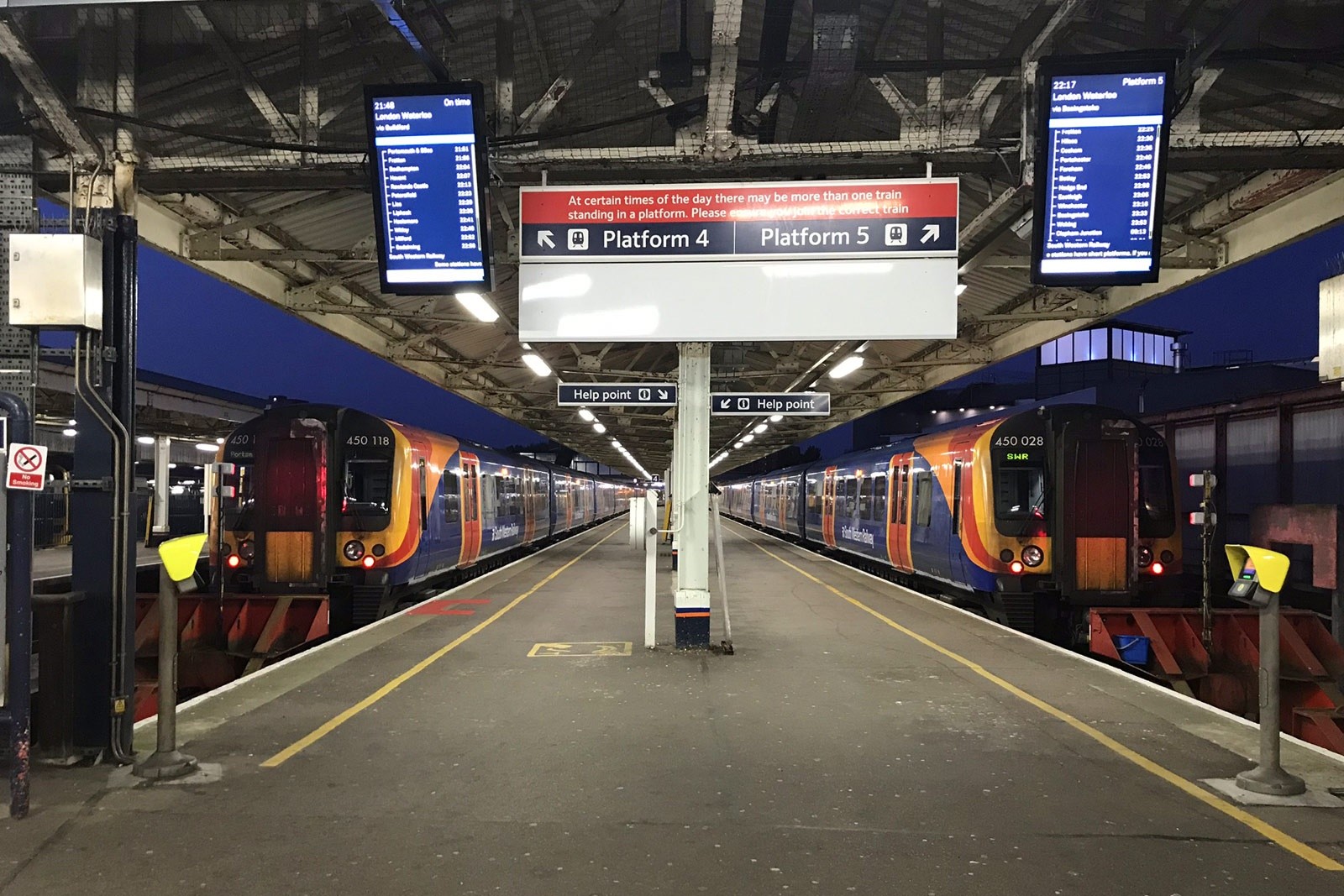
[707,401,1182,646]
[206,401,646,635]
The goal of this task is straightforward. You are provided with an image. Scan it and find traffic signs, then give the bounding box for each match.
[710,392,832,415]
[558,382,678,407]
[519,183,960,256]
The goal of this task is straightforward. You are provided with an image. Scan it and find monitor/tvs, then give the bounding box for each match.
[1031,49,1176,287]
[363,79,496,296]
